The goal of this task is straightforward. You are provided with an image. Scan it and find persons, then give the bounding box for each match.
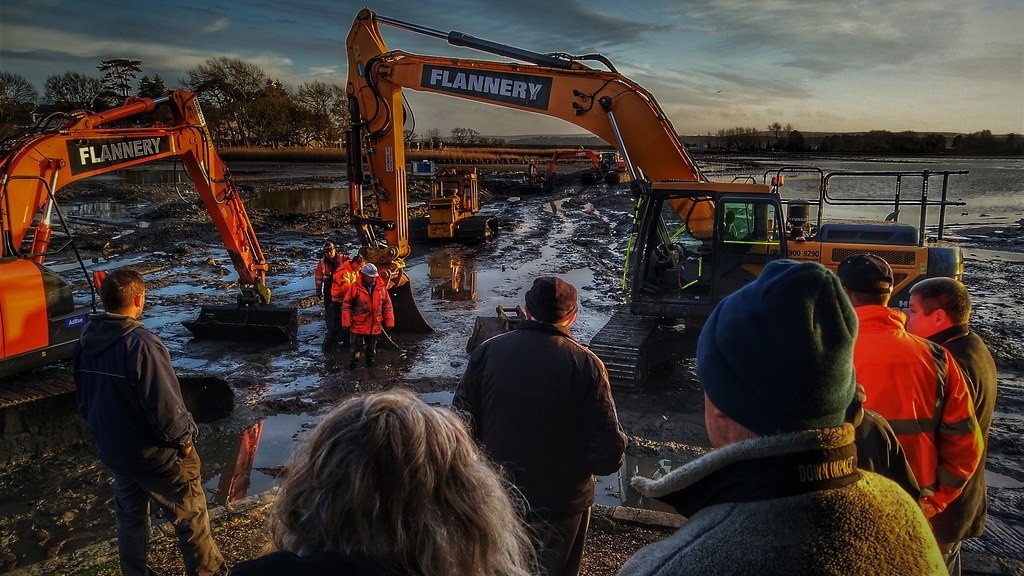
[449,276,628,576]
[314,242,395,368]
[229,389,534,576]
[71,269,229,576]
[615,252,998,576]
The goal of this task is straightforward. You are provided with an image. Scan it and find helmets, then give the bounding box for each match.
[361,263,379,278]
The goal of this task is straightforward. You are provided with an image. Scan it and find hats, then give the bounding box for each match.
[524,277,579,324]
[837,252,894,295]
[695,258,861,438]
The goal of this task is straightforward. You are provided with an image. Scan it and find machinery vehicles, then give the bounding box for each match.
[342,6,964,400]
[0,87,299,411]
[205,420,264,507]
[408,140,634,252]
[425,252,478,302]
